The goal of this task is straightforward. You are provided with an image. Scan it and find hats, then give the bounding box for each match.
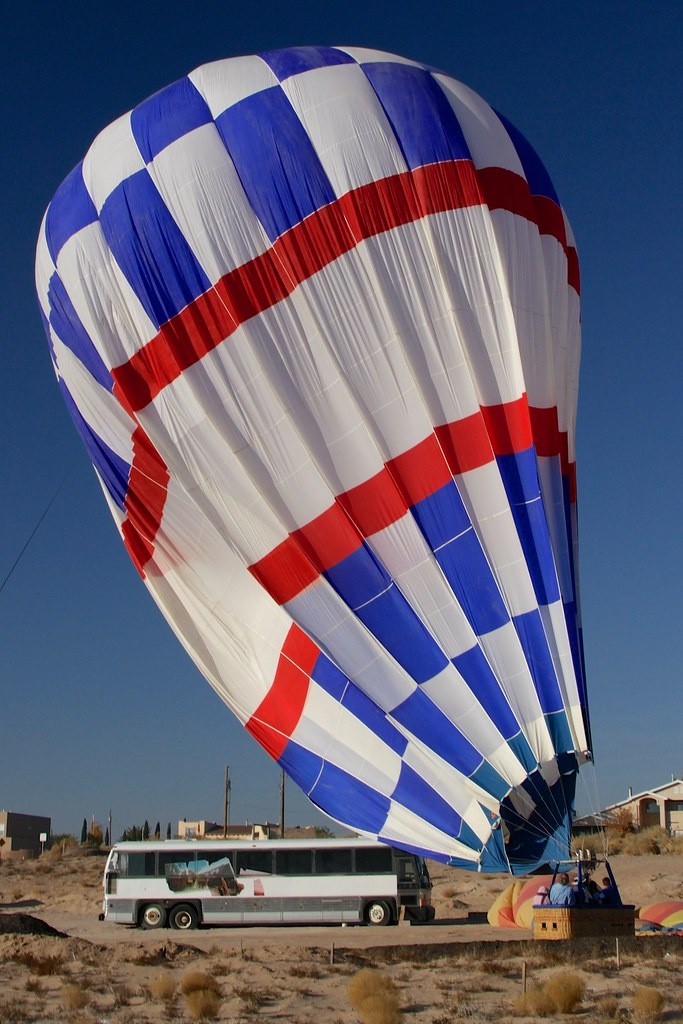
[571,877,586,886]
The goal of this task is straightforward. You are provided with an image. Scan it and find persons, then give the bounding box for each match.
[550,874,575,906]
[582,874,604,906]
[571,877,593,906]
[533,886,552,906]
[599,878,620,906]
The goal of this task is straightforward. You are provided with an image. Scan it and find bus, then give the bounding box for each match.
[96,836,438,929]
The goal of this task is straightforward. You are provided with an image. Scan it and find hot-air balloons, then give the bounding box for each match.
[484,870,682,939]
[35,42,638,942]
